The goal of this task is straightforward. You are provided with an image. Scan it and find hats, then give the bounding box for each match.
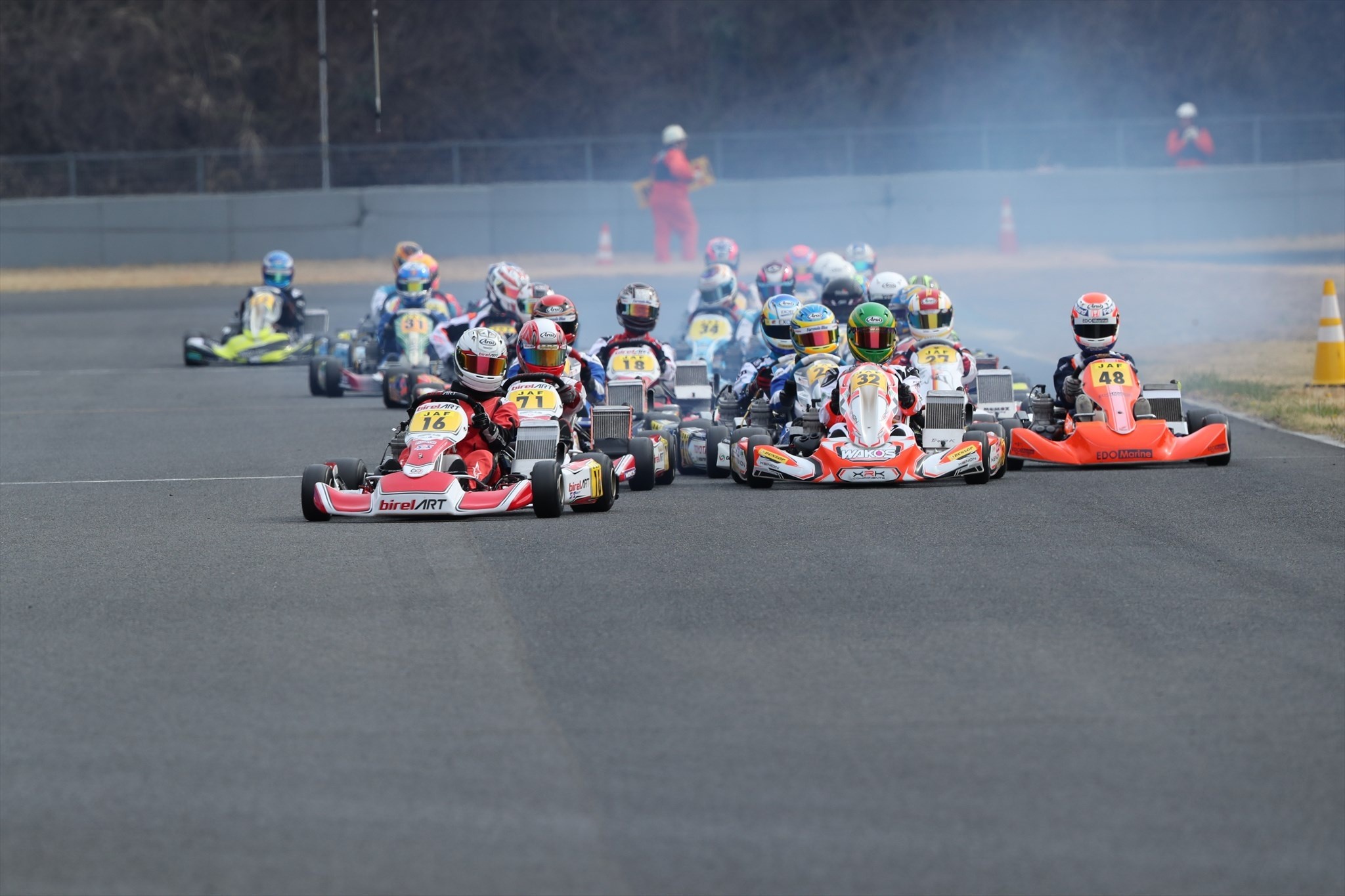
[662,124,687,147]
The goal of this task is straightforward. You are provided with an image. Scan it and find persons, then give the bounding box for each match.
[588,282,677,404]
[648,123,705,262]
[239,250,306,341]
[1053,292,1151,434]
[1167,102,1212,167]
[688,236,977,438]
[370,241,606,449]
[381,327,519,491]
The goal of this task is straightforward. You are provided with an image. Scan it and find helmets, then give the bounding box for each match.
[532,295,578,347]
[699,263,737,306]
[261,250,294,290]
[517,318,567,383]
[761,294,802,353]
[488,264,530,314]
[756,262,796,302]
[786,240,942,333]
[1071,292,1120,353]
[453,327,508,393]
[515,282,555,324]
[407,253,440,293]
[394,242,423,277]
[789,303,840,357]
[615,283,660,333]
[908,289,953,340]
[704,237,740,274]
[846,303,898,364]
[396,263,432,307]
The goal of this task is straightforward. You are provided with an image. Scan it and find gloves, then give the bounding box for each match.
[831,389,840,406]
[472,402,491,428]
[1062,376,1082,398]
[556,384,576,404]
[897,383,911,400]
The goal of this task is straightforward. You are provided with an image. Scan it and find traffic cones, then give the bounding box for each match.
[596,225,615,267]
[1305,279,1345,387]
[997,198,1019,257]
